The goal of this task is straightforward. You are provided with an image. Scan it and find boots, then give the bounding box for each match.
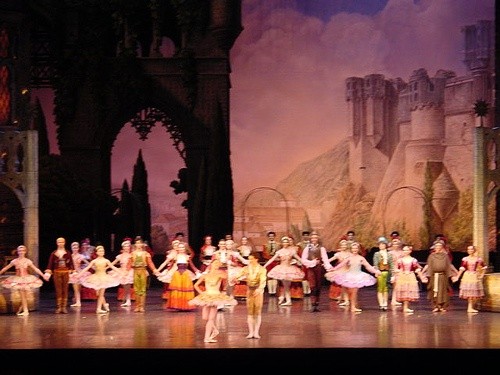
[56,298,62,313]
[254,315,261,338]
[246,315,254,339]
[134,295,140,312]
[140,295,146,312]
[267,280,276,296]
[62,299,68,314]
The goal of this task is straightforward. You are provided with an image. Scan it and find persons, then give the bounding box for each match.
[232,251,267,339]
[0,228,488,316]
[187,258,240,342]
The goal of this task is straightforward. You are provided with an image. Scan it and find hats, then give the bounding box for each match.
[374,237,389,244]
[310,232,320,238]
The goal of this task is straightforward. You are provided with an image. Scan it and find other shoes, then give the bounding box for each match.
[404,308,414,312]
[71,303,81,306]
[203,339,217,343]
[278,296,284,305]
[121,302,132,306]
[339,301,350,305]
[97,310,107,313]
[315,303,322,312]
[439,307,447,312]
[351,307,362,312]
[310,302,317,312]
[281,302,292,306]
[18,310,29,315]
[210,330,219,339]
[468,309,479,313]
[432,307,439,312]
[104,303,110,311]
[391,301,402,305]
